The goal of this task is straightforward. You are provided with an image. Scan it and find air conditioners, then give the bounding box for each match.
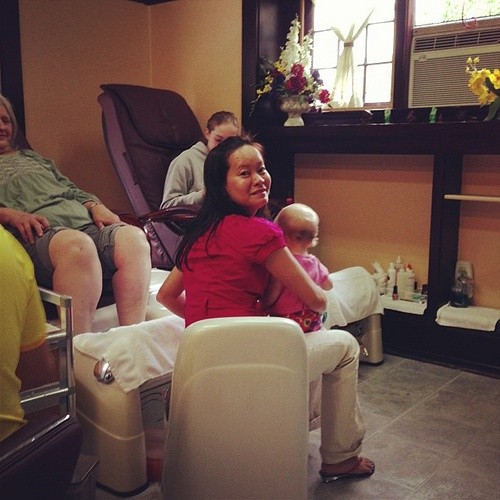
[408,14,500,109]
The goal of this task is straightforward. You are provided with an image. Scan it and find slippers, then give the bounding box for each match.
[318,456,375,484]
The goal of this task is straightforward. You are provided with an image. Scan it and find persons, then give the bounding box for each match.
[260,203,333,332]
[0,95,153,336]
[156,134,376,483]
[160,111,242,210]
[0,224,83,442]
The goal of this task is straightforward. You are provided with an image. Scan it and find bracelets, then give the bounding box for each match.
[89,201,102,211]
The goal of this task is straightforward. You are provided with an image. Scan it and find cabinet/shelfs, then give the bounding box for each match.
[242,116,500,377]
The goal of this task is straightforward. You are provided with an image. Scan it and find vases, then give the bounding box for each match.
[279,95,308,126]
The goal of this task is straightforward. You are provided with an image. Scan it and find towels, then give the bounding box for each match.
[380,294,427,316]
[318,262,387,329]
[72,312,187,392]
[435,298,500,331]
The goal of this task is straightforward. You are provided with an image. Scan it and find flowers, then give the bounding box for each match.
[464,57,500,121]
[249,13,332,116]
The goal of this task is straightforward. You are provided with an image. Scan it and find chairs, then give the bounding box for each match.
[159,315,309,500]
[97,83,285,272]
